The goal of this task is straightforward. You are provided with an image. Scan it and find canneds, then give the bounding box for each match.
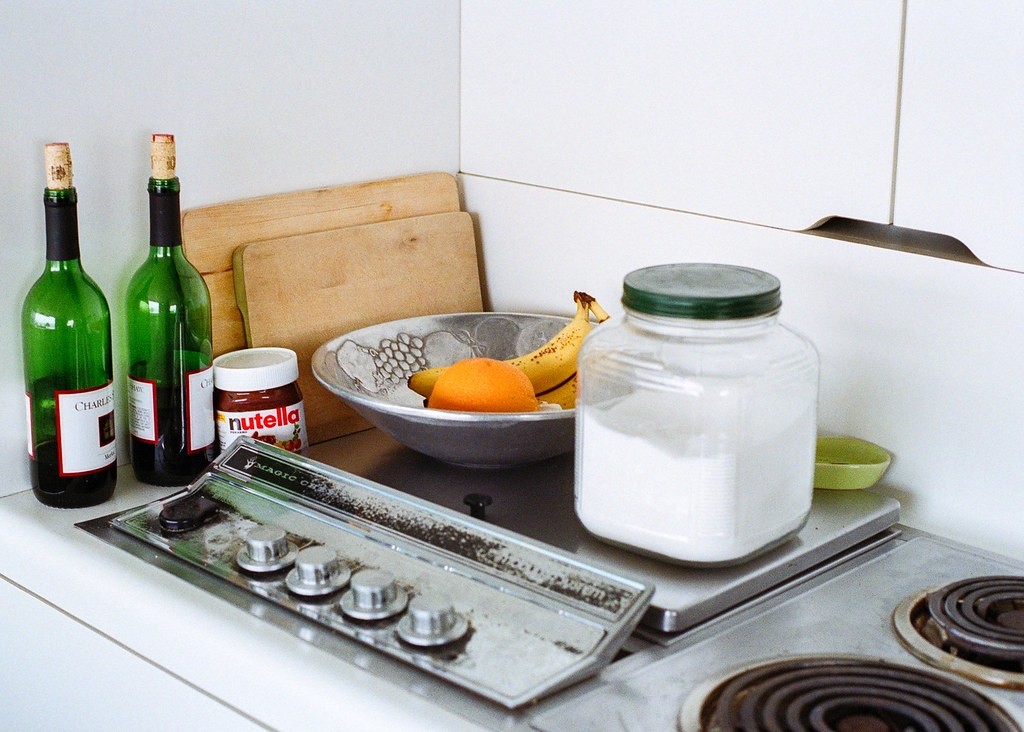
[213,347,309,456]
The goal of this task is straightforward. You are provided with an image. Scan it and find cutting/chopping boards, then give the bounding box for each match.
[233,210,484,444]
[180,171,461,359]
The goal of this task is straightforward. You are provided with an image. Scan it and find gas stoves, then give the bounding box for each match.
[519,523,1024,732]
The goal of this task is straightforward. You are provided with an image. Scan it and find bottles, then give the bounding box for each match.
[574,263,821,570]
[123,134,215,486]
[21,142,116,508]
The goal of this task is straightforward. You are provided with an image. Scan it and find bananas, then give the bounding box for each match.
[407,290,611,409]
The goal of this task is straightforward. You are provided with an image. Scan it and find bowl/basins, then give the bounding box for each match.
[310,312,598,467]
[816,436,892,490]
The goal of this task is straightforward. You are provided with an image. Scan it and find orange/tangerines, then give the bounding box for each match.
[426,357,538,413]
